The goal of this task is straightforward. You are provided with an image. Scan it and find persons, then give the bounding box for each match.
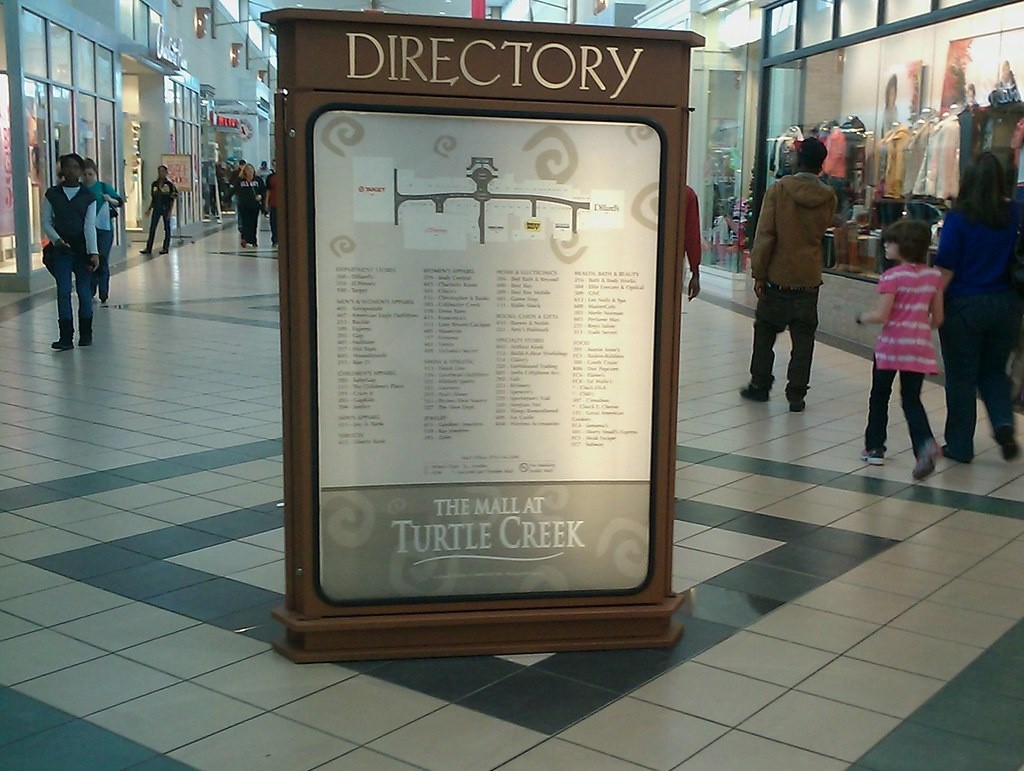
[685,185,702,301]
[139,165,178,254]
[740,137,837,413]
[202,156,278,247]
[882,75,897,137]
[42,154,122,350]
[768,117,865,269]
[872,104,961,273]
[853,146,1024,479]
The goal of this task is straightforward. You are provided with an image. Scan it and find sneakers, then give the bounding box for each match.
[860,449,884,467]
[911,440,942,479]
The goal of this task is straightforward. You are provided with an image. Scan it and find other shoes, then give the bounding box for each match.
[789,400,807,412]
[996,432,1018,459]
[139,249,152,254]
[940,446,974,463]
[740,385,770,401]
[99,298,109,307]
[159,248,168,254]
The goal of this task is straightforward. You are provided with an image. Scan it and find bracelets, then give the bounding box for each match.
[855,316,861,325]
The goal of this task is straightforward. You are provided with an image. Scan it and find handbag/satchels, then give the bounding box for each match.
[260,203,269,217]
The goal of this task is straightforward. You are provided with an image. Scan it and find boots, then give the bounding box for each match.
[78,315,93,346]
[51,320,75,350]
